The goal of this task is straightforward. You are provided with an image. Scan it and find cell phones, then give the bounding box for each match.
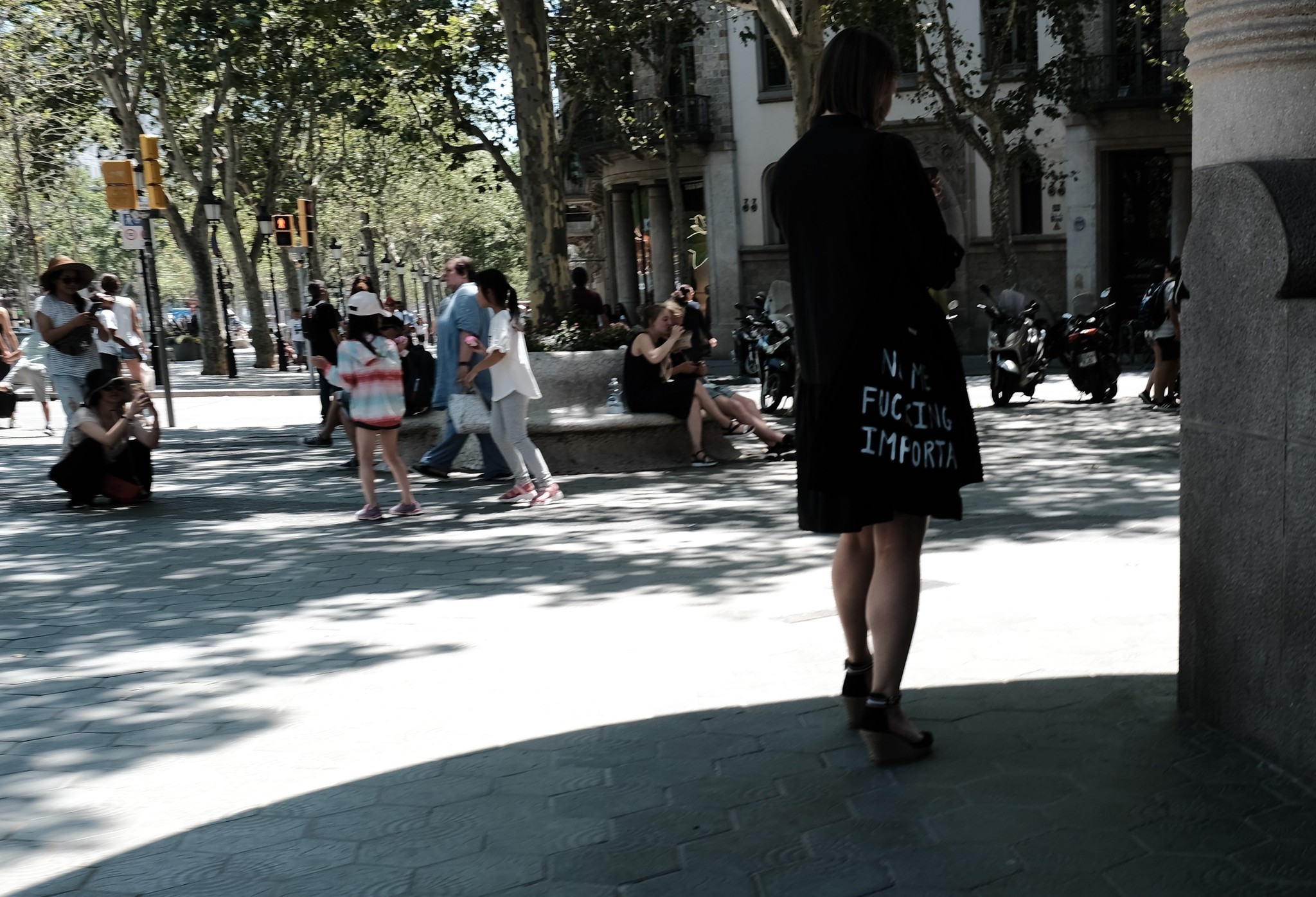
[129,383,144,397]
[90,301,102,315]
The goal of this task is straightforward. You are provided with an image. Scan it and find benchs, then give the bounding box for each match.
[401,347,696,473]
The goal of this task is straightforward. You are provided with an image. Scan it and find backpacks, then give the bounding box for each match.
[301,300,327,341]
[1136,278,1176,330]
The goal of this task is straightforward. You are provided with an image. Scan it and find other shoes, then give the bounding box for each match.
[766,432,798,456]
[479,472,515,480]
[335,455,360,469]
[10,419,22,427]
[1137,390,1180,411]
[354,504,382,520]
[387,499,424,515]
[409,461,451,481]
[297,433,334,449]
[44,425,55,436]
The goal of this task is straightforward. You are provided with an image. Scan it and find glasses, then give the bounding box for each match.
[59,273,83,285]
[378,326,396,331]
[101,381,126,392]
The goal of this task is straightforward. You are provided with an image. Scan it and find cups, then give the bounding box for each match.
[668,326,681,340]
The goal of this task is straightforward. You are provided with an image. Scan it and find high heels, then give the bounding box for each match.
[841,654,873,730]
[863,695,936,766]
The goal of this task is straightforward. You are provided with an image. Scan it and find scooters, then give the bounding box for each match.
[975,283,1048,408]
[733,279,800,413]
[1053,286,1129,402]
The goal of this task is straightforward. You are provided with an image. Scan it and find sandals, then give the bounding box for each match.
[498,482,537,502]
[690,450,719,467]
[721,418,756,439]
[530,482,564,506]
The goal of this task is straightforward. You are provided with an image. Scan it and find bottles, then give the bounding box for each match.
[606,378,625,414]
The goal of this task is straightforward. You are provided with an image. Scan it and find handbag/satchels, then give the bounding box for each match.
[448,387,491,434]
[138,362,156,391]
[48,322,94,355]
[101,476,141,505]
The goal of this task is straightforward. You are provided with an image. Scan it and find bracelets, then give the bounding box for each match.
[456,361,469,366]
[121,414,133,421]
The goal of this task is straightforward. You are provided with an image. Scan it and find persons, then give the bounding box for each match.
[1137,257,1187,412]
[770,27,985,766]
[288,254,562,520]
[0,255,161,511]
[572,267,799,468]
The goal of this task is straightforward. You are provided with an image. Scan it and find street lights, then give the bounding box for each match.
[329,240,448,346]
[202,184,242,378]
[255,205,289,372]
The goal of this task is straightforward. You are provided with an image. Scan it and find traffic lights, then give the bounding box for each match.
[297,199,316,247]
[271,214,295,247]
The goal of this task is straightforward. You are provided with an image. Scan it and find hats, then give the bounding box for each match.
[39,255,95,291]
[347,291,392,317]
[82,368,135,401]
